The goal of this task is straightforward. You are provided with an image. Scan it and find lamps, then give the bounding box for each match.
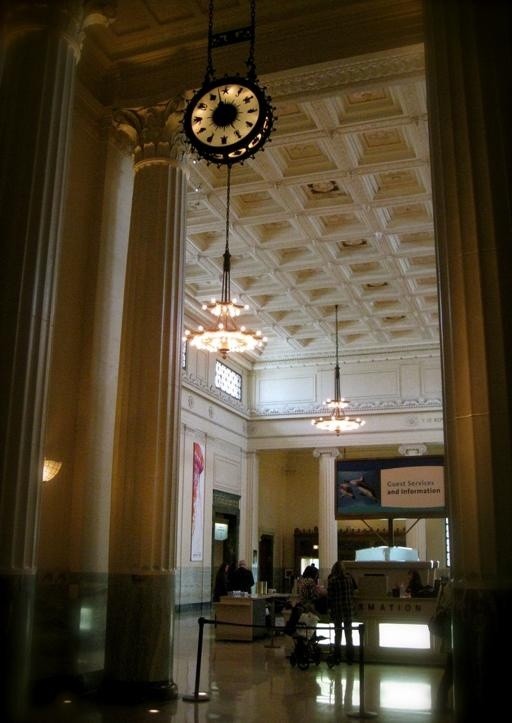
[312,304,366,434]
[181,158,270,360]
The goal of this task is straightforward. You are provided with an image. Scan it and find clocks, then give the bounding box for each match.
[180,0,277,166]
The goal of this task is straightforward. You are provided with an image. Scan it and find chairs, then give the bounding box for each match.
[280,606,292,623]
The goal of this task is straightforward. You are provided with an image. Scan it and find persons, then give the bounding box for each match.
[227,560,254,594]
[302,563,319,584]
[404,569,420,596]
[213,561,229,602]
[327,561,359,664]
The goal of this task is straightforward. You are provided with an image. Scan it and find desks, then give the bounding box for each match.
[216,594,292,642]
